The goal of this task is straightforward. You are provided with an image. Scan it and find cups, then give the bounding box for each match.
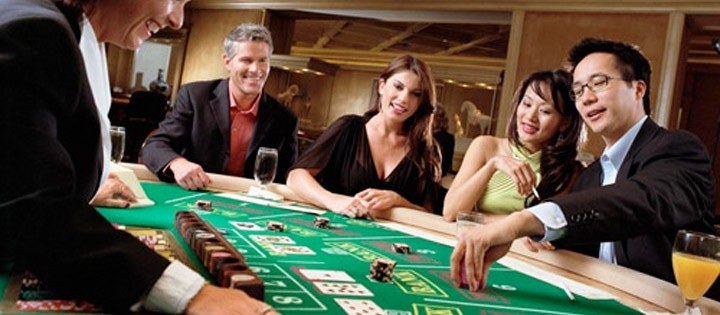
[110,127,125,163]
[456,210,485,242]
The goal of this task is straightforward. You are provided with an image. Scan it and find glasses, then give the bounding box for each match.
[569,73,635,101]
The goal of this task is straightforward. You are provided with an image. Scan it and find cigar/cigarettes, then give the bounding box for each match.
[532,186,540,200]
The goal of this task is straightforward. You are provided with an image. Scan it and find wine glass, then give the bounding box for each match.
[254,147,278,200]
[672,229,720,315]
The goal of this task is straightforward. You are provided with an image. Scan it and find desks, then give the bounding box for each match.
[0,163,720,315]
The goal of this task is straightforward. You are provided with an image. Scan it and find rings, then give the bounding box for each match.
[119,185,124,194]
[259,305,272,315]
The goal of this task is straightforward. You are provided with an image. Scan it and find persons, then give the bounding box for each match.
[126,79,169,163]
[140,22,298,191]
[443,69,584,252]
[450,37,720,302]
[431,102,456,178]
[0,0,282,315]
[286,54,442,220]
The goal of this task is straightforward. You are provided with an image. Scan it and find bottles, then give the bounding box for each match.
[150,69,168,93]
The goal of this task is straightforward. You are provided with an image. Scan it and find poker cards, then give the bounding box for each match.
[311,281,375,297]
[331,297,387,315]
[298,269,357,283]
[229,220,264,232]
[248,235,297,245]
[266,245,317,255]
